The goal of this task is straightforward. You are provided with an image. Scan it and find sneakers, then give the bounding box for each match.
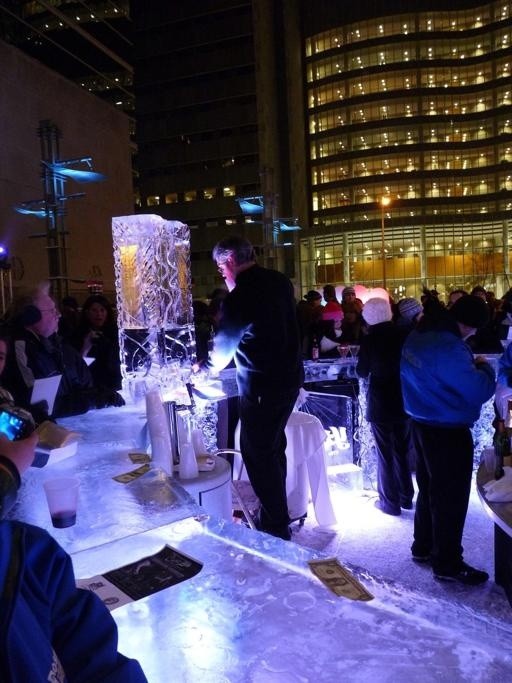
[411,545,489,585]
[374,500,413,516]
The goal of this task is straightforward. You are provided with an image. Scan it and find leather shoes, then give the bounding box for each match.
[242,510,258,528]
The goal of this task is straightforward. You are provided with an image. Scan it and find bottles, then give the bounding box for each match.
[310,338,319,361]
[496,419,510,456]
[503,398,512,455]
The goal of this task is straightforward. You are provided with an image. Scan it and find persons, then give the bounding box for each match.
[191,233,304,541]
[0,518,148,683]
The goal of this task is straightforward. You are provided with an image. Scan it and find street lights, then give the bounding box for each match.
[377,192,393,291]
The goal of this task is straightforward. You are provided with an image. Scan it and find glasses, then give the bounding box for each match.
[40,307,56,313]
[217,267,224,273]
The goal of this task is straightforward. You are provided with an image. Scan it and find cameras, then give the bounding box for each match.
[1,405,35,443]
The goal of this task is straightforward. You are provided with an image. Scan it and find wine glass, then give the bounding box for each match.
[348,344,361,363]
[336,343,350,363]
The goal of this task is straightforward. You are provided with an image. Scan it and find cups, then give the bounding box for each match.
[42,477,82,529]
[482,449,497,473]
[144,390,207,481]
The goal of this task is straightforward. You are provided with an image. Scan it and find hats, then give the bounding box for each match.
[303,291,322,301]
[449,295,489,329]
[361,298,393,325]
[342,288,355,295]
[398,298,424,319]
